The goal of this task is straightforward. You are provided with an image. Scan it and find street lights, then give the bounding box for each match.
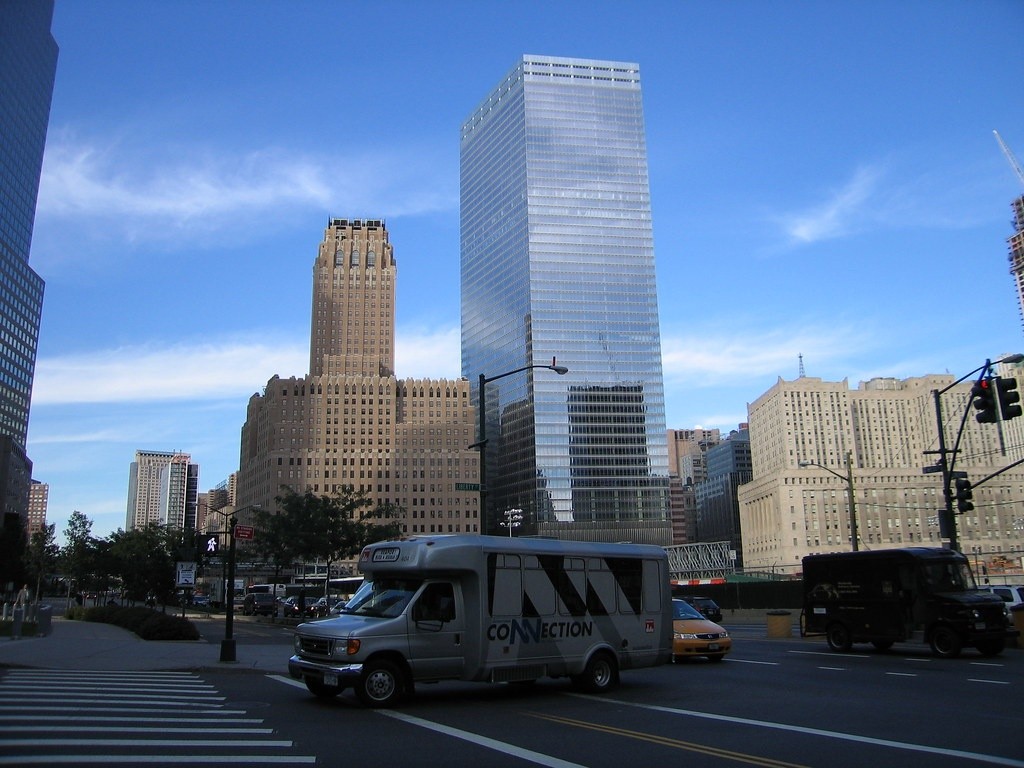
[796,461,860,551]
[500,507,523,537]
[216,515,242,663]
[932,352,1024,550]
[184,497,262,612]
[478,363,570,535]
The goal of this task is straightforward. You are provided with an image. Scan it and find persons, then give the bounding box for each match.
[15,584,32,606]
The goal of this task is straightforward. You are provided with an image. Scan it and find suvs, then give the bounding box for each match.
[244,593,277,616]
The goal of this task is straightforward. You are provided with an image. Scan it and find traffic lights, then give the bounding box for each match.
[971,380,997,424]
[955,480,974,513]
[200,535,220,557]
[996,376,1023,419]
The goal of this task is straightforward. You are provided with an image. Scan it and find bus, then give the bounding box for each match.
[248,584,287,598]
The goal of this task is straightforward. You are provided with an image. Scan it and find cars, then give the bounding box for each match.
[284,596,331,620]
[86,592,97,600]
[192,596,208,607]
[234,598,244,611]
[316,597,349,614]
[144,596,157,607]
[680,596,722,622]
[672,598,730,663]
[977,584,1024,626]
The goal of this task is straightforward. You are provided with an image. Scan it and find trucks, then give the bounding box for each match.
[288,530,670,708]
[800,545,1011,660]
